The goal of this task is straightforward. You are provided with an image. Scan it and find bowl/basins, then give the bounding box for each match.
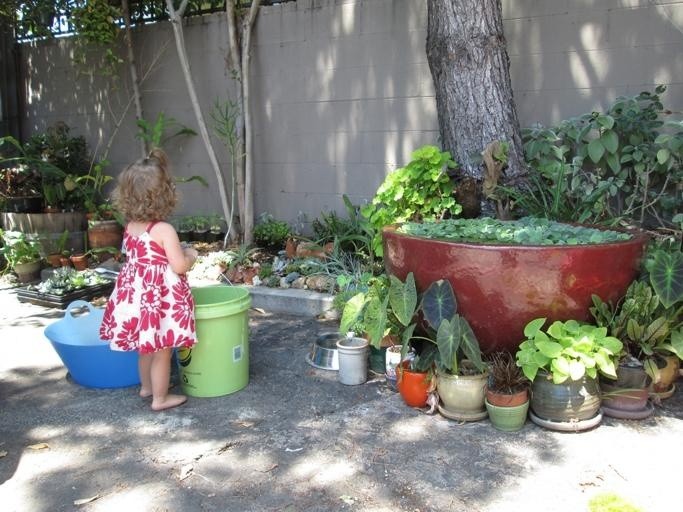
[303,331,341,372]
[45,300,175,386]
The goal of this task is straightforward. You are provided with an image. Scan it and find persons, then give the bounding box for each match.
[98,155,198,412]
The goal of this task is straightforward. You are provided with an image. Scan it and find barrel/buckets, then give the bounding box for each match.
[45,302,144,389]
[174,260,254,398]
[335,337,369,386]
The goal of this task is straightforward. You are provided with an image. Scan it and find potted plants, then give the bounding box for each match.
[0,132,123,312]
